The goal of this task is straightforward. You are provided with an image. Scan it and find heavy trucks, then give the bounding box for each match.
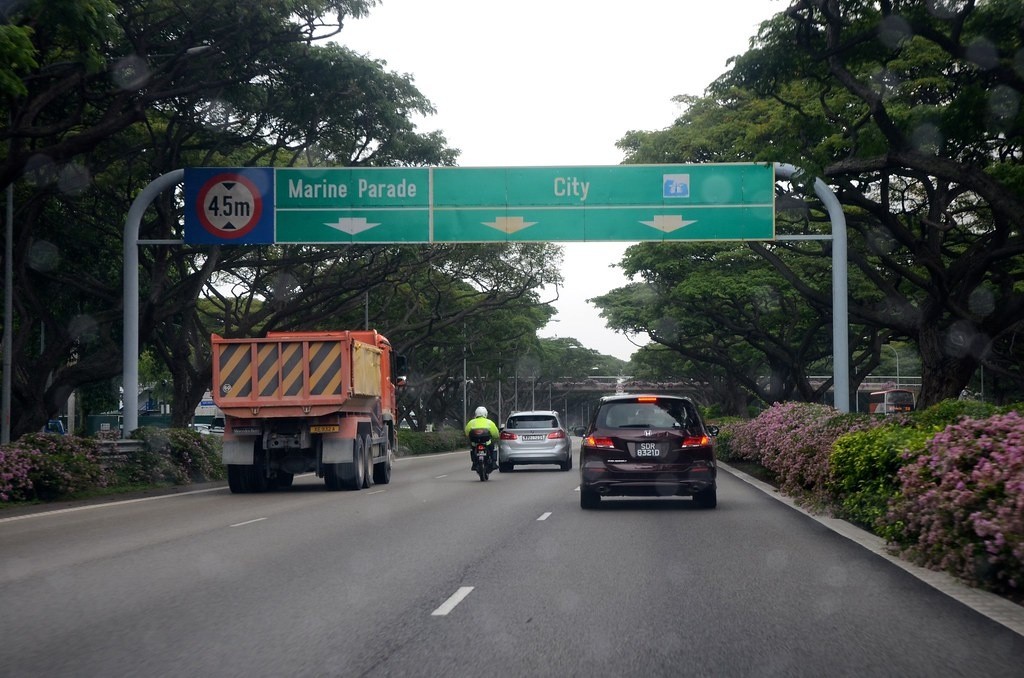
[209,329,409,494]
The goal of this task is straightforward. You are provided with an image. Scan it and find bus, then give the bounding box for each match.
[869,389,915,414]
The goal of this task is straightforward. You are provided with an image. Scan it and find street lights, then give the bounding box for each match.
[881,344,899,389]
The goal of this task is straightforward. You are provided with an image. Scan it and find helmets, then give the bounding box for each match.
[475,406,488,418]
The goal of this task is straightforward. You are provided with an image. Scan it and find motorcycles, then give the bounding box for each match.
[468,429,498,482]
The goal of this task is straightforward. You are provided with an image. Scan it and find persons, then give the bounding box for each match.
[960,386,971,400]
[464,406,500,470]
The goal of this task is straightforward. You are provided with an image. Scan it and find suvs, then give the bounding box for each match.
[573,393,720,511]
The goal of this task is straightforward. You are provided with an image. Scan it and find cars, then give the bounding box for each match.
[497,410,574,474]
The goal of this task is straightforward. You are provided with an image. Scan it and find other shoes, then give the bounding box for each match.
[493,463,499,469]
[471,465,478,470]
